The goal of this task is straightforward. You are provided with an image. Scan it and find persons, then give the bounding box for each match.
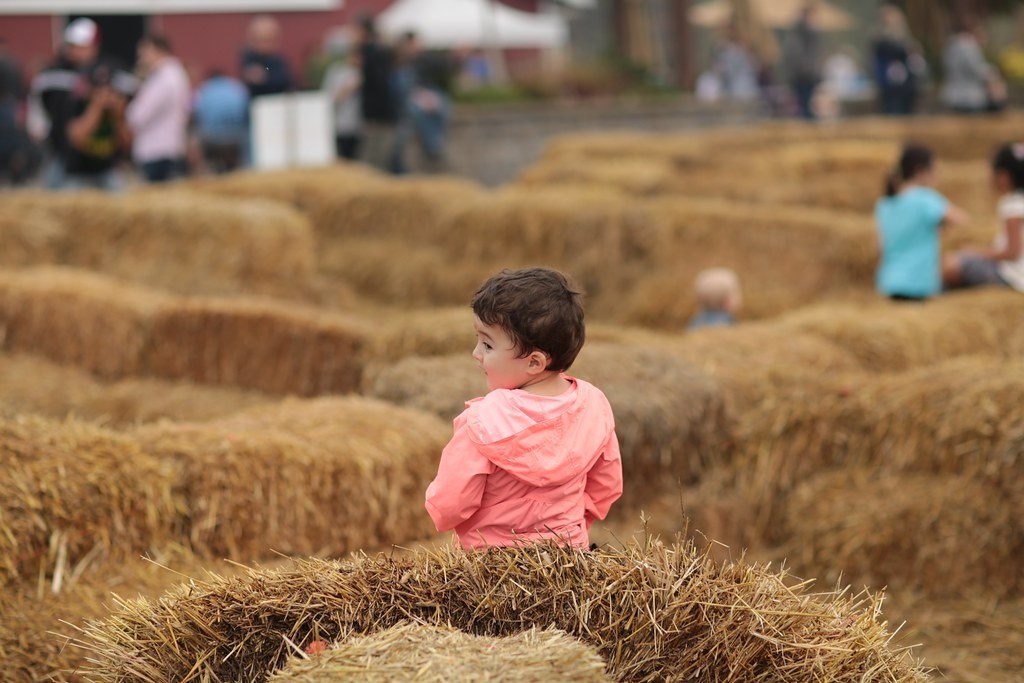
[0,39,41,184]
[699,31,827,121]
[935,28,1007,114]
[38,17,128,187]
[873,141,963,301]
[425,268,623,551]
[240,14,300,94]
[945,141,1024,292]
[126,33,192,180]
[192,72,249,169]
[873,6,925,111]
[321,15,488,173]
[688,267,740,330]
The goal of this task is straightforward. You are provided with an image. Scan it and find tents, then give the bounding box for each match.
[372,0,568,87]
